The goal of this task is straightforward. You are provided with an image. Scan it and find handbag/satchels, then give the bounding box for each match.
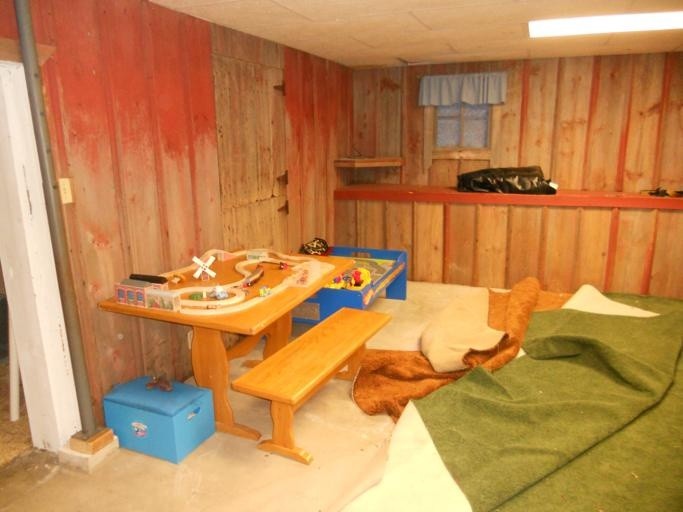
[456,166,556,194]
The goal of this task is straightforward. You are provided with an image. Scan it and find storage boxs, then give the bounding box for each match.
[106,375,212,463]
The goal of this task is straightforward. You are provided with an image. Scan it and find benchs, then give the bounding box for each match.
[223,303,396,464]
